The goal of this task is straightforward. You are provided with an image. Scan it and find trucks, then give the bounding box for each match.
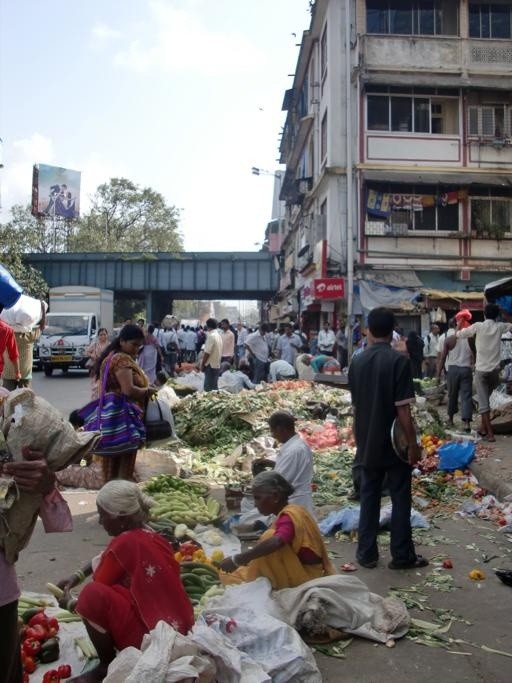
[40,285,115,374]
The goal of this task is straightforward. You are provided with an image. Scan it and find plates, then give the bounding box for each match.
[391,416,421,462]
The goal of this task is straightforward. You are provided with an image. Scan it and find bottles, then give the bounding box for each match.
[224,513,241,531]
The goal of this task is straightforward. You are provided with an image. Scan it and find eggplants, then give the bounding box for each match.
[306,399,338,420]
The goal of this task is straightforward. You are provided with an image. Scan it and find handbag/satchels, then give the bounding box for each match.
[167,342,178,352]
[85,357,97,369]
[77,392,147,455]
[489,390,511,412]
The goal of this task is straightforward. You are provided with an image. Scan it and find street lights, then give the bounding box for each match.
[253,164,282,254]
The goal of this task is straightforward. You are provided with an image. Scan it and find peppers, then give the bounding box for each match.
[143,474,207,493]
[174,544,224,564]
[19,608,72,683]
[420,435,442,455]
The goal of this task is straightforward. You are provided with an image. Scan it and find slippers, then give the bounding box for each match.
[387,553,429,568]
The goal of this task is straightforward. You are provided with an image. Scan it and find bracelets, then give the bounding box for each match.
[231,553,241,568]
[66,598,77,614]
[15,369,21,374]
[73,568,87,584]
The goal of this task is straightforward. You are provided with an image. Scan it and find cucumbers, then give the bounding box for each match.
[141,519,178,538]
[179,561,221,610]
[150,491,221,527]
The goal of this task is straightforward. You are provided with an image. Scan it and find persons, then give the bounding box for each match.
[88,327,111,357]
[209,470,338,593]
[132,315,364,392]
[393,304,512,444]
[0,302,23,384]
[54,184,72,216]
[53,477,192,682]
[344,309,404,501]
[1,386,58,682]
[346,304,429,569]
[2,301,47,391]
[43,182,68,215]
[93,323,160,476]
[229,408,319,541]
